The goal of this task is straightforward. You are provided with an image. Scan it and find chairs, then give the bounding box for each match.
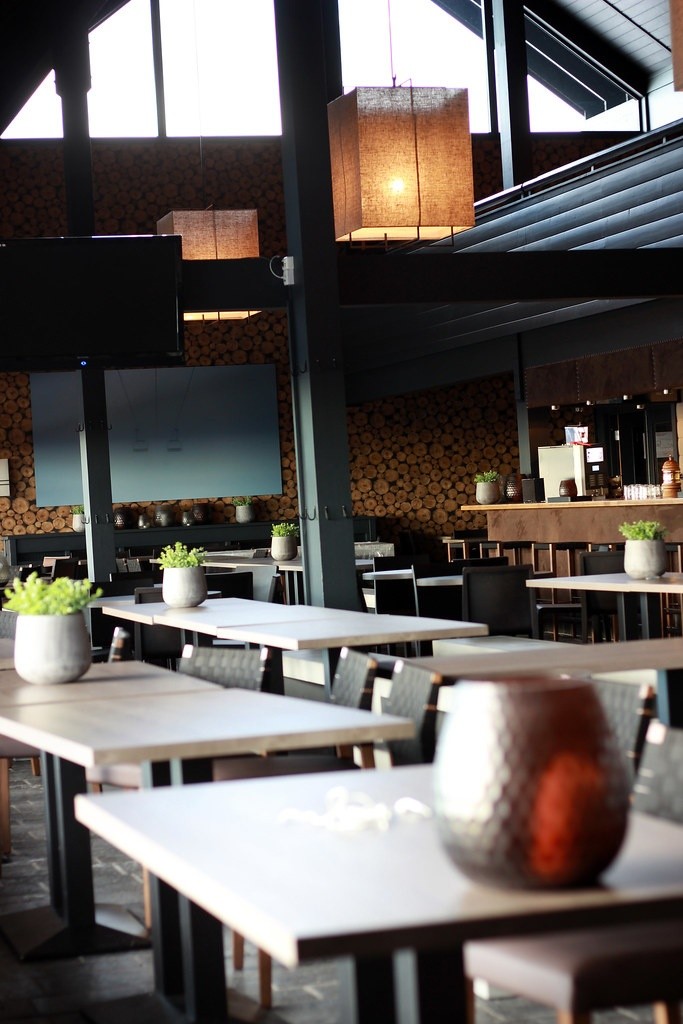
[0,556,683,1024]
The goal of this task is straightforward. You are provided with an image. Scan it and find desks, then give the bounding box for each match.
[0,498,683,1024]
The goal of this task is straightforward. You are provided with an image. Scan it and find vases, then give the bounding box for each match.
[192,504,210,524]
[433,675,628,887]
[113,507,134,530]
[153,505,175,527]
[138,515,151,529]
[505,474,527,503]
[180,510,195,526]
[559,480,577,497]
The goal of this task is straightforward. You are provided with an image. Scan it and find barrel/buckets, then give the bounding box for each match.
[503,474,525,502]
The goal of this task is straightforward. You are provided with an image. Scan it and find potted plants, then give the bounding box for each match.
[157,541,207,607]
[71,505,85,532]
[619,520,669,579]
[474,471,502,504]
[271,523,300,561]
[3,571,103,683]
[231,496,254,523]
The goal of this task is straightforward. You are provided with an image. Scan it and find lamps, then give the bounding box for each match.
[327,0,475,250]
[157,0,262,321]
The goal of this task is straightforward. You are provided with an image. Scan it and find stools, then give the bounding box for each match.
[448,541,683,645]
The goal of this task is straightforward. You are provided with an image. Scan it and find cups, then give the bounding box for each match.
[433,674,628,887]
[559,478,577,497]
